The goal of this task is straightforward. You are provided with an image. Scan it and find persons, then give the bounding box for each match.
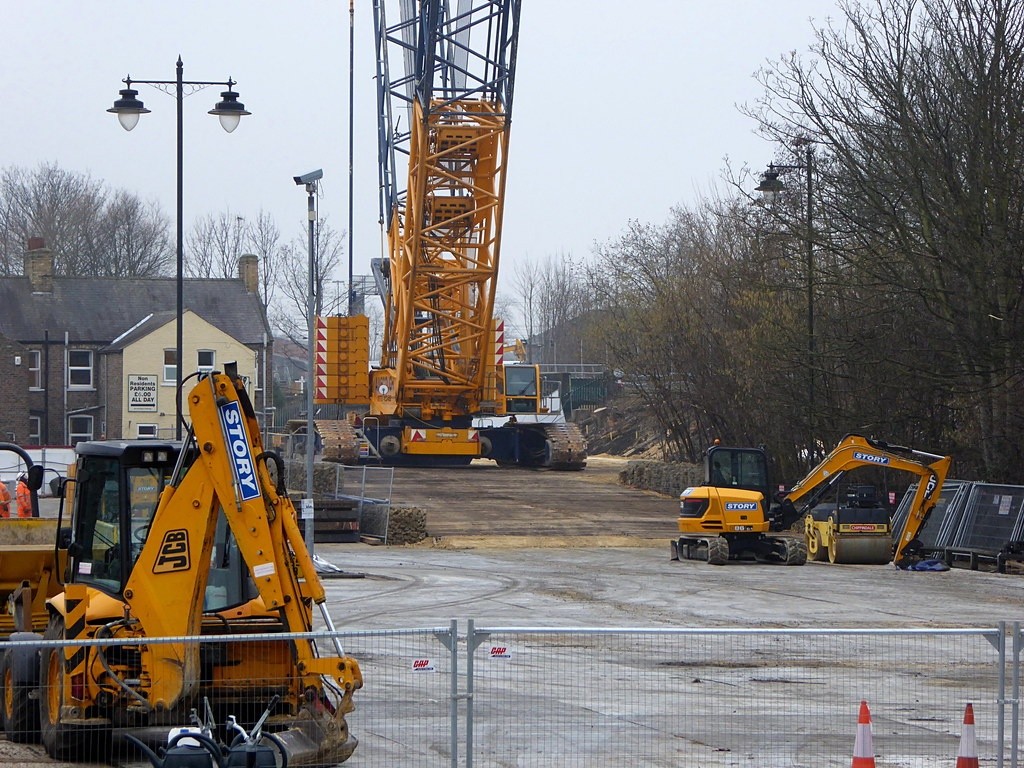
[0,482,12,518]
[16,471,32,517]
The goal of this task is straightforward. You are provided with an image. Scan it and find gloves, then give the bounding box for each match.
[24,507,30,512]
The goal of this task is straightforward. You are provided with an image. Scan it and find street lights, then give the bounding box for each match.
[105,55,252,441]
[753,145,814,475]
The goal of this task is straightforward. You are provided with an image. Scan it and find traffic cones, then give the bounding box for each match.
[956,704,981,768]
[852,700,877,768]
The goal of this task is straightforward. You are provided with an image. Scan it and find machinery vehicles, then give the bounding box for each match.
[667,429,951,569]
[279,1,595,470]
[0,361,366,768]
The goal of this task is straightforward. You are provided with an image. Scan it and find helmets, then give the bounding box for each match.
[16,472,25,480]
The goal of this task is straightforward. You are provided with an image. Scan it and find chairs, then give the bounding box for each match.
[714,461,739,488]
